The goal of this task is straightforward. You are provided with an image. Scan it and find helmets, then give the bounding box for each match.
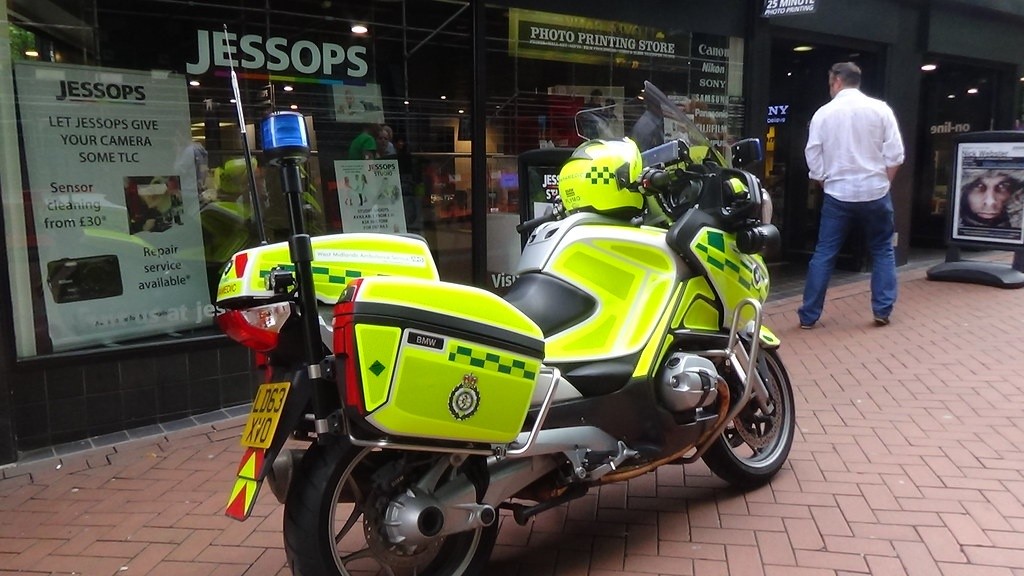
[559,138,645,216]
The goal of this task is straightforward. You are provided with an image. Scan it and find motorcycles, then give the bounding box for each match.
[82,155,325,277]
[215,21,797,576]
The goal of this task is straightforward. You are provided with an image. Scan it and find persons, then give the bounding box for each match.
[169,134,209,223]
[959,168,1024,238]
[343,174,369,206]
[798,62,904,328]
[631,99,731,153]
[337,89,382,114]
[349,114,396,160]
[584,89,619,140]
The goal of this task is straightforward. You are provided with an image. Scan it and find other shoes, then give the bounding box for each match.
[875,316,889,325]
[802,325,813,329]
